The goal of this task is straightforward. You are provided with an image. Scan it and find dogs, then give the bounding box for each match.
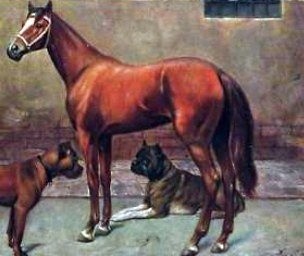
[110,139,246,224]
[0,139,84,256]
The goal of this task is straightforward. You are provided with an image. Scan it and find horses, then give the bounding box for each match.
[5,1,258,256]
